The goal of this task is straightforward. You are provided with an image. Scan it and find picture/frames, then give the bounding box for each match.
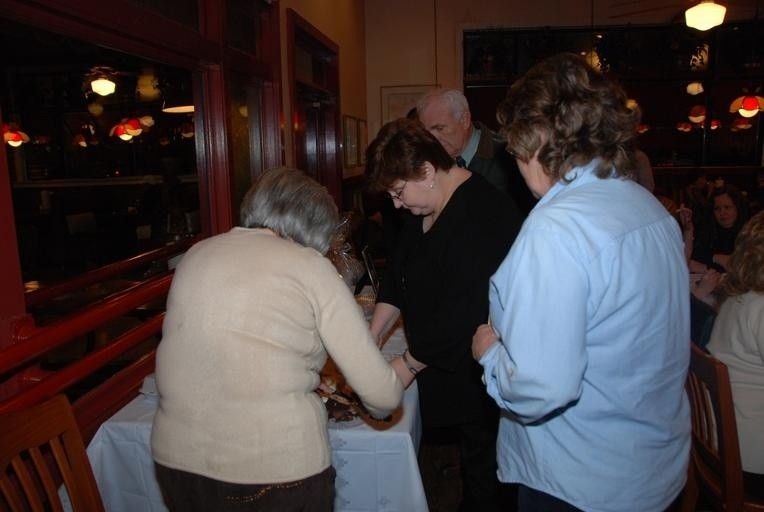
[380,84,441,128]
[341,113,369,169]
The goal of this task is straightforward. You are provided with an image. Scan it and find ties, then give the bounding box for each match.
[455,156,467,168]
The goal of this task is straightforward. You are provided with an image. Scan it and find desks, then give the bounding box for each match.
[101,298,423,512]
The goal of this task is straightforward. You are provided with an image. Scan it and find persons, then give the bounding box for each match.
[361,48,764,512]
[149,165,407,512]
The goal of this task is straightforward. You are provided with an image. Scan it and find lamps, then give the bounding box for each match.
[728,93,763,119]
[112,118,144,143]
[2,128,28,148]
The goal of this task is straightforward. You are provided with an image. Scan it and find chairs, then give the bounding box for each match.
[1,394,106,512]
[677,343,764,510]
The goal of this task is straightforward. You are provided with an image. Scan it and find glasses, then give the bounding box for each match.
[388,179,408,201]
